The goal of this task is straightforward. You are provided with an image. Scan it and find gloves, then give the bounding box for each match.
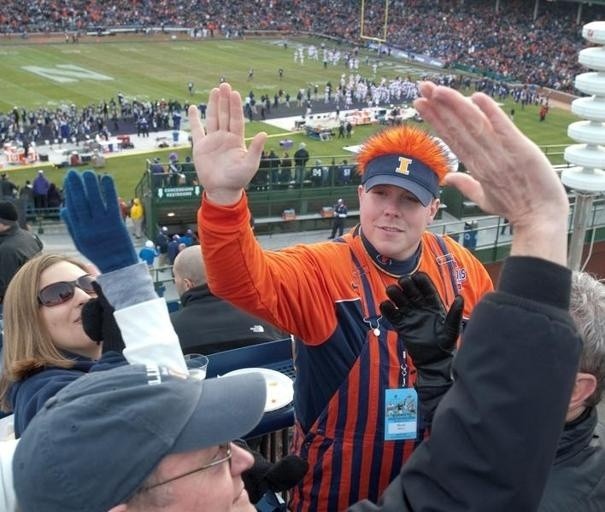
[380,272,463,387]
[60,170,139,273]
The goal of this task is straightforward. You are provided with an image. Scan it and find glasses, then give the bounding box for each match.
[145,442,232,488]
[38,274,96,306]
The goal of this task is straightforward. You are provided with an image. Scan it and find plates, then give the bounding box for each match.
[219,366,294,414]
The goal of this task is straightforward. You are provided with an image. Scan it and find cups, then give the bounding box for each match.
[182,353,208,381]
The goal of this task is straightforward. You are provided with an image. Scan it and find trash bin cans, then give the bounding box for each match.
[464,220,478,250]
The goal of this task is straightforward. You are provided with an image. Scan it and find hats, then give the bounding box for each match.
[13,366,266,512]
[361,154,440,207]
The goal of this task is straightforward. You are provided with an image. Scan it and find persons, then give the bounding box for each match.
[166,244,291,356]
[1,0,605,295]
[1,169,188,438]
[6,81,574,512]
[186,78,495,512]
[378,264,605,512]
